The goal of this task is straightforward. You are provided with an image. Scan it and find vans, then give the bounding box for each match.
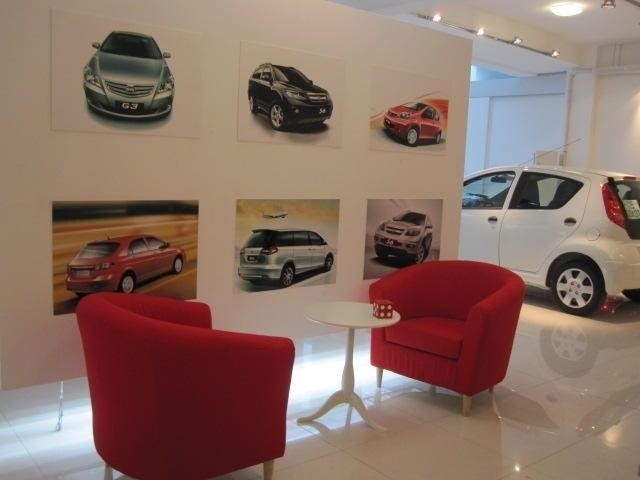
[238,227,336,288]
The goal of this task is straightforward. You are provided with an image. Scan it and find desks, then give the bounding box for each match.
[298,301,402,433]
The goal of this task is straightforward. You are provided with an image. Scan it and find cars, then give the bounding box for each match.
[457,165,640,317]
[383,102,443,147]
[488,307,640,439]
[65,234,187,298]
[80,30,175,121]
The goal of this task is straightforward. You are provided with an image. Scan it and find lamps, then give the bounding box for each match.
[411,11,560,59]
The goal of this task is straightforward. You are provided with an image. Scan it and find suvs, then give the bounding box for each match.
[374,210,434,266]
[248,62,333,131]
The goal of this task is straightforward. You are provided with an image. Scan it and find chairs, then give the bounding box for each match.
[76,292,295,480]
[369,261,525,417]
